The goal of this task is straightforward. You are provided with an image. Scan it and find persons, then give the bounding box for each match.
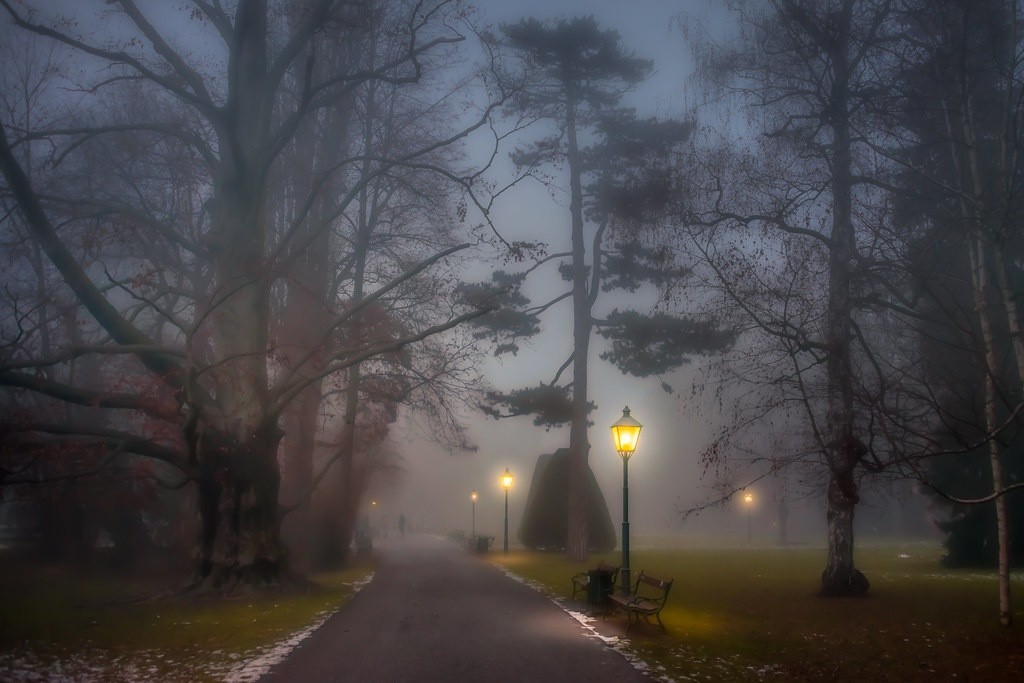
[398,514,406,537]
[776,498,789,544]
[585,564,615,616]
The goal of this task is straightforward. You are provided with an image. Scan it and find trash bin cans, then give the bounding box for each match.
[477,536,489,551]
[586,569,614,614]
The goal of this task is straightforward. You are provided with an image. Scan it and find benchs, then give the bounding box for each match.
[570,560,622,603]
[464,532,496,551]
[602,569,674,634]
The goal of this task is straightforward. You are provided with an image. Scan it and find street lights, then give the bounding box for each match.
[470,489,479,541]
[499,467,513,556]
[743,492,754,547]
[610,405,644,593]
